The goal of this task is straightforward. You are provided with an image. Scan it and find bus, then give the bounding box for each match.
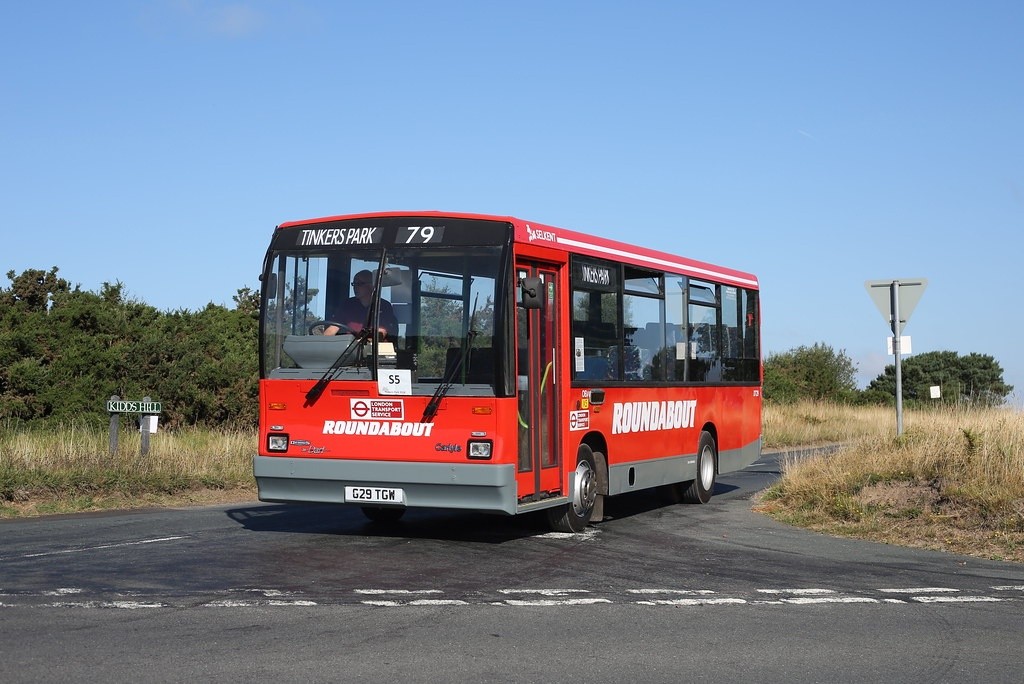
[252,217,763,532]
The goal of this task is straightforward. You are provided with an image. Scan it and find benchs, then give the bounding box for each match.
[445,321,757,385]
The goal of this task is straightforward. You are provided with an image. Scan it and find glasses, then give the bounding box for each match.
[351,282,364,286]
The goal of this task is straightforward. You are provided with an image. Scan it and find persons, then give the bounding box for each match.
[324,270,399,344]
